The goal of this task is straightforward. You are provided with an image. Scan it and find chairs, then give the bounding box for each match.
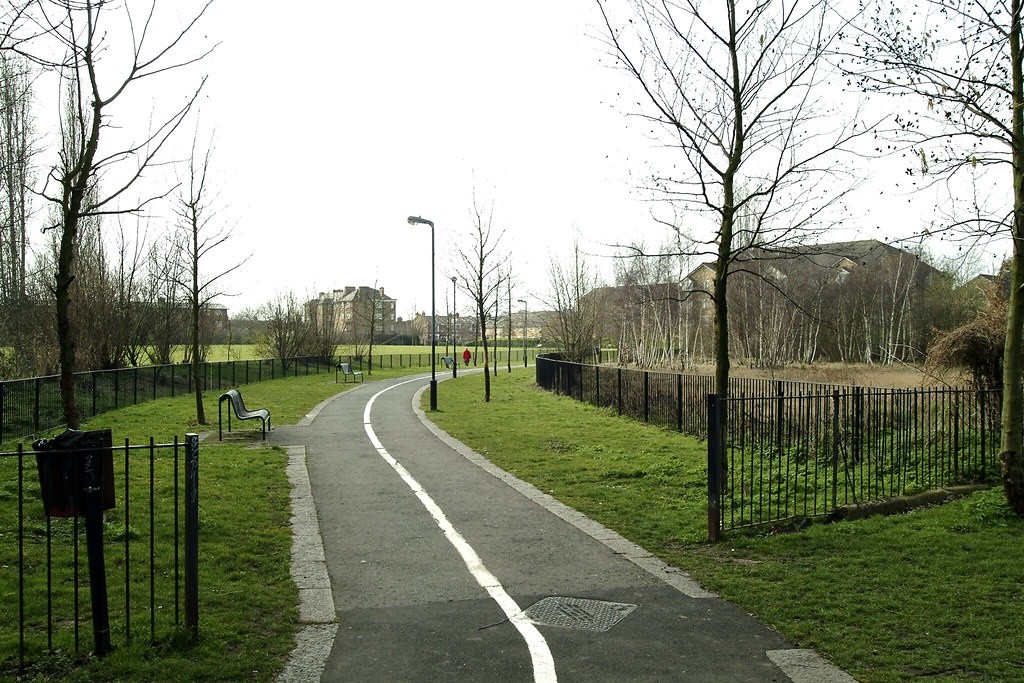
[336,363,363,383]
[441,357,460,370]
[218,389,270,441]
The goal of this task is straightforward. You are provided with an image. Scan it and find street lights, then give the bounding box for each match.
[451,276,460,378]
[517,300,529,368]
[407,215,439,410]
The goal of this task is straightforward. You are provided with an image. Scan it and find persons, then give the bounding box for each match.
[462,348,471,366]
